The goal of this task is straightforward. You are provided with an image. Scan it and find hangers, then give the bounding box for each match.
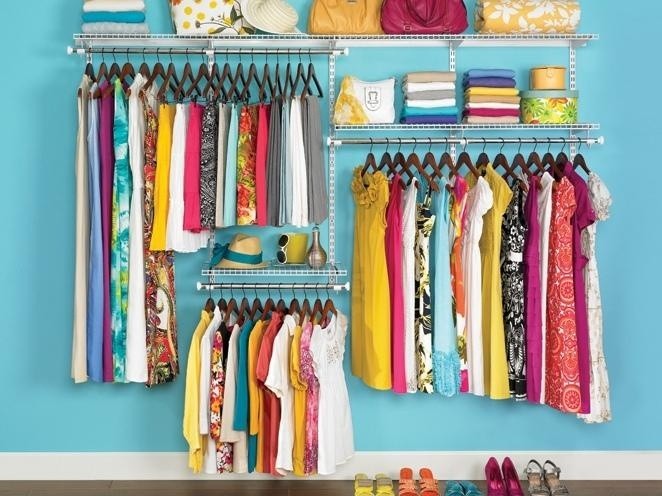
[359,135,592,192]
[77,45,324,103]
[203,280,338,328]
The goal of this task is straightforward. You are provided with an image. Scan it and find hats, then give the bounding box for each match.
[208,233,268,269]
[241,0,302,35]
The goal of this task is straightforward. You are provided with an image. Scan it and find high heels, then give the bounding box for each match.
[544,461,569,496]
[355,473,374,496]
[420,468,439,496]
[526,460,550,496]
[502,457,524,496]
[399,468,418,496]
[485,457,506,496]
[375,474,395,496]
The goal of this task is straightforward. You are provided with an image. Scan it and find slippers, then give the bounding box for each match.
[444,480,465,496]
[459,480,482,496]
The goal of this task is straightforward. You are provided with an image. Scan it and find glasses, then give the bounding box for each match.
[276,233,289,264]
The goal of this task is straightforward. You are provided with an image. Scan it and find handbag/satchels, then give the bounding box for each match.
[380,0,468,34]
[307,0,385,35]
[333,77,395,124]
